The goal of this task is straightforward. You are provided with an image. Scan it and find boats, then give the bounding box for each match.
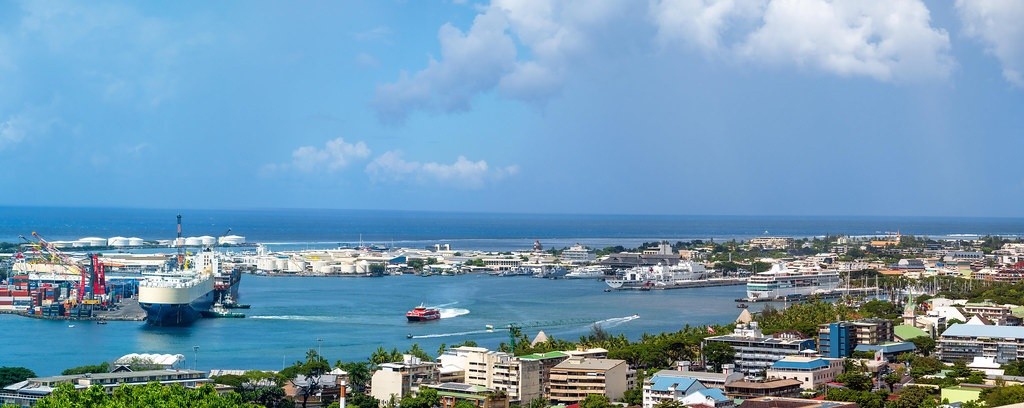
[407,333,412,338]
[200,292,250,318]
[218,291,251,309]
[406,307,440,321]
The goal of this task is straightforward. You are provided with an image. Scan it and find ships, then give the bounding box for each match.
[138,214,215,326]
